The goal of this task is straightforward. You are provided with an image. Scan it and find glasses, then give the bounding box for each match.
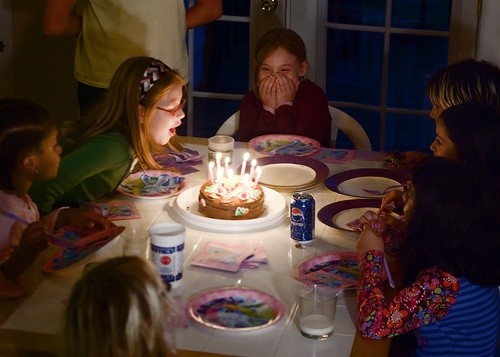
[157,99,187,118]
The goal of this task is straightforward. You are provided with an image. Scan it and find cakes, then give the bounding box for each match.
[198,178,265,220]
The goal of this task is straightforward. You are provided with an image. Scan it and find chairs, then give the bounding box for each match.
[215,105,372,152]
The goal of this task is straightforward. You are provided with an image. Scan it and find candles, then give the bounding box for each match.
[215,153,222,183]
[209,161,215,186]
[249,159,257,184]
[224,156,230,178]
[240,152,249,179]
[257,166,262,184]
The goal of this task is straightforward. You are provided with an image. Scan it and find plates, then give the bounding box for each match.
[249,134,321,156]
[317,198,407,232]
[186,285,285,332]
[323,168,410,198]
[116,169,190,200]
[236,156,329,192]
[42,225,125,274]
[173,183,289,233]
[296,252,360,290]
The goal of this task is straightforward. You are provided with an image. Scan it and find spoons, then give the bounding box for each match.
[361,183,410,195]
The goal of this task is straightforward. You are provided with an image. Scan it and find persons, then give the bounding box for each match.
[0,96,113,300]
[25,57,187,218]
[237,26,333,148]
[41,0,222,117]
[427,59,499,122]
[62,255,180,356]
[428,104,499,160]
[355,155,499,356]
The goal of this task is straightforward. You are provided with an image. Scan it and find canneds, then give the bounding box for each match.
[289,192,315,243]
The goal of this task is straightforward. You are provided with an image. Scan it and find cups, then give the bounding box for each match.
[149,222,185,289]
[297,284,338,340]
